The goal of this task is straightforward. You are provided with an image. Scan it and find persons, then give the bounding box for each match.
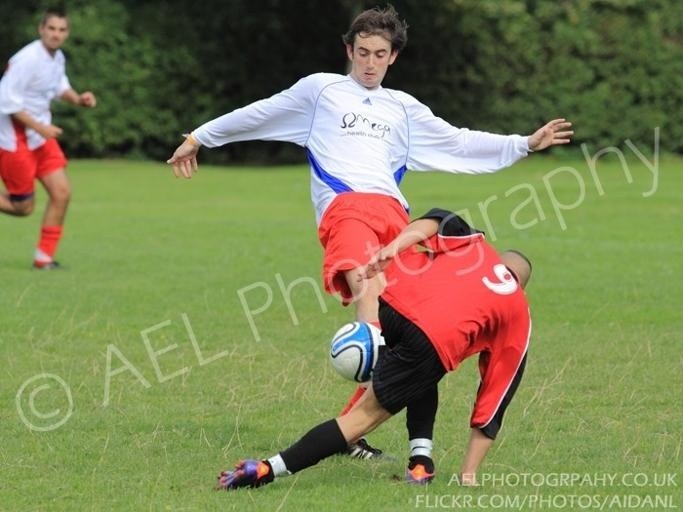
[216,207,532,488]
[1,8,96,270]
[166,3,573,461]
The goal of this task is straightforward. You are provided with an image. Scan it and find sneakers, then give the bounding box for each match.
[406,455,435,484]
[214,459,275,492]
[336,438,383,460]
[33,259,60,270]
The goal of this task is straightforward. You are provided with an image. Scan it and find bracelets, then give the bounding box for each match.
[181,131,203,149]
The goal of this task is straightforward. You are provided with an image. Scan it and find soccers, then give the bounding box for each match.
[329,321,386,383]
